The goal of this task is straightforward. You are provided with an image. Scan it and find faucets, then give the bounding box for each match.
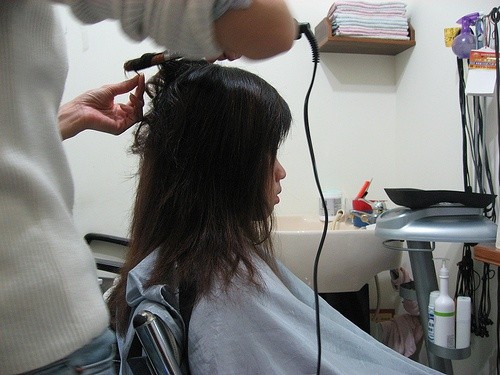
[348,199,388,224]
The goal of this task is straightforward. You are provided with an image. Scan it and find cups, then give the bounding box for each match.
[318,188,347,221]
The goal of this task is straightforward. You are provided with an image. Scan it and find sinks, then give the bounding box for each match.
[250,214,403,293]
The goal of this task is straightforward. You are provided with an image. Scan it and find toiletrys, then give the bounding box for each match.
[433,256,456,349]
[427,290,440,344]
[455,296,471,349]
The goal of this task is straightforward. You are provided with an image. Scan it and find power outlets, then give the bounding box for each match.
[370,309,395,322]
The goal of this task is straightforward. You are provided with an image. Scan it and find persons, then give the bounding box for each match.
[101,58,446,374]
[2,0,296,375]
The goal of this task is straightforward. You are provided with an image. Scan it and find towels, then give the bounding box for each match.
[326,1,409,40]
[377,313,425,358]
